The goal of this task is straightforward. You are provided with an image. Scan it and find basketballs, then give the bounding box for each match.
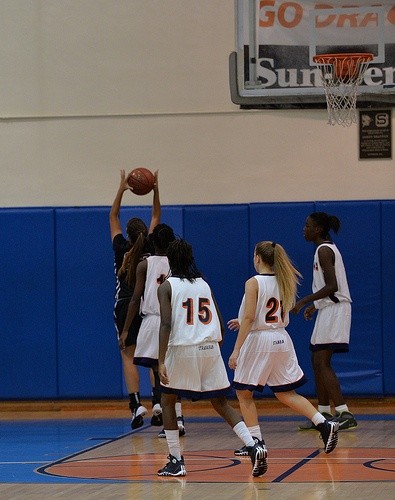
[126,168,154,195]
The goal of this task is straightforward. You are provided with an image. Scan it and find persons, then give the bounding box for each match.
[290,211,358,433]
[228,240,339,456]
[157,238,268,477]
[109,168,186,438]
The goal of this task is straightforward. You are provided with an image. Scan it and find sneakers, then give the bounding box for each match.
[330,410,358,431]
[129,403,148,430]
[156,454,187,476]
[234,436,266,455]
[150,402,163,426]
[158,414,185,437]
[250,443,268,477]
[297,411,334,431]
[318,419,339,453]
